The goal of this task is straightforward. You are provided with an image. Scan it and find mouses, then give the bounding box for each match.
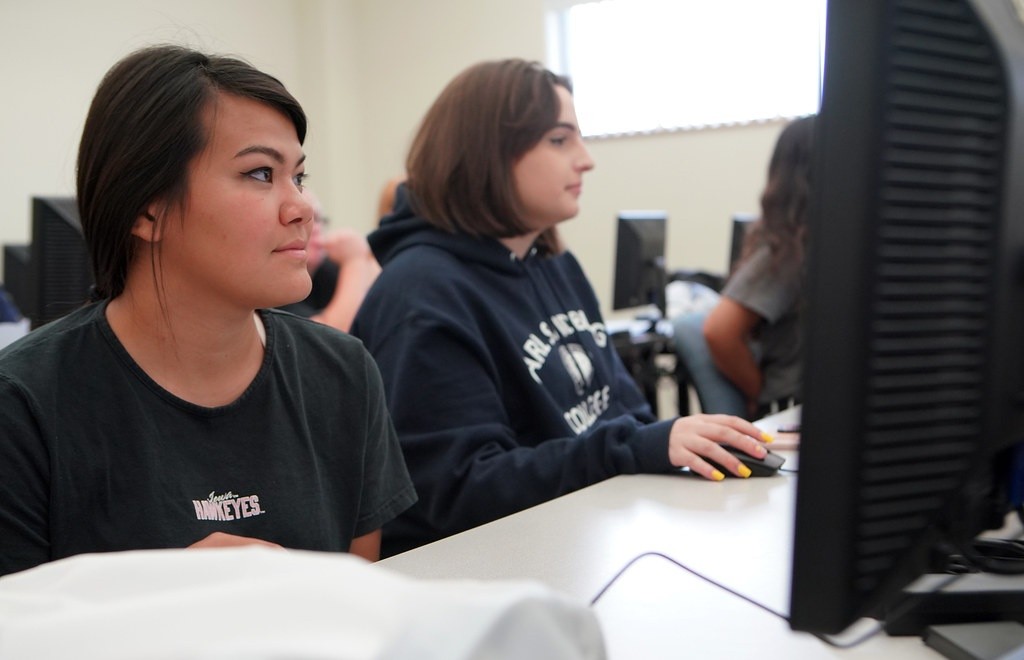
[691,445,785,478]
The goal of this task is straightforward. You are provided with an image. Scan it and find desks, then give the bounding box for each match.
[370,404,1024,660]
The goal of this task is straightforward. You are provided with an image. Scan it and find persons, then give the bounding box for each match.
[0,47,417,578]
[271,60,821,563]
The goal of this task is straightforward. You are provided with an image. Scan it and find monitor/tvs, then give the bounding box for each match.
[30,197,97,329]
[730,218,762,278]
[613,211,667,310]
[789,0,1024,660]
